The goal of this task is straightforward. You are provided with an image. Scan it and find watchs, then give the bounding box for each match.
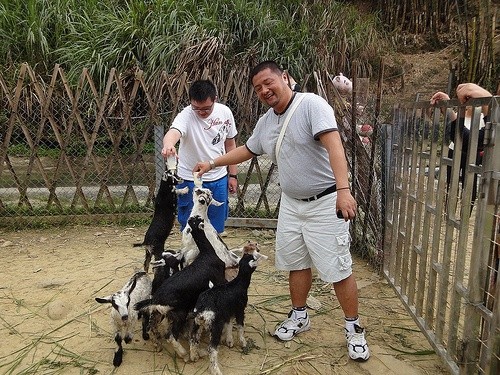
[229,174,238,180]
[208,159,215,169]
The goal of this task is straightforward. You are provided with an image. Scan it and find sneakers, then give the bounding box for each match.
[274,310,311,341]
[344,323,370,361]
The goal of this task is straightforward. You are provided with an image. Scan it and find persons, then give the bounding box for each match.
[430,76,500,220]
[191,60,370,361]
[161,79,239,235]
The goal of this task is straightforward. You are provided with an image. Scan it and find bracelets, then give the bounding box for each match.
[336,187,350,191]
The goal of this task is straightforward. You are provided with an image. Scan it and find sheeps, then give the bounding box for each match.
[95,170,269,375]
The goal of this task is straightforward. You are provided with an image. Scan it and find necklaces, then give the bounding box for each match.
[277,113,281,125]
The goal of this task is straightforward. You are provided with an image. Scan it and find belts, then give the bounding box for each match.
[301,184,336,201]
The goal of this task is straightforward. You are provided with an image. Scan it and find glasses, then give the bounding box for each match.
[192,103,213,112]
